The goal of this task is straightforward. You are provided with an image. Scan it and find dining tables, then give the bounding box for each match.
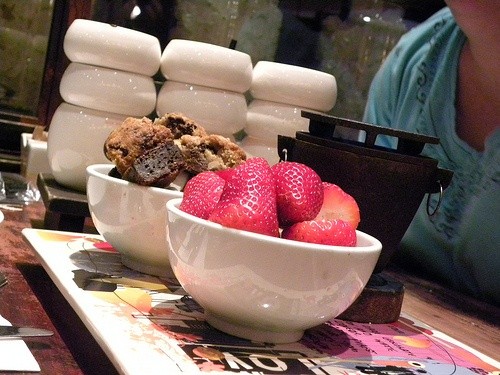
[0,200,500,375]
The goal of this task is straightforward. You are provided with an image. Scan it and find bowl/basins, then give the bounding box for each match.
[86,163,184,278]
[165,198,382,344]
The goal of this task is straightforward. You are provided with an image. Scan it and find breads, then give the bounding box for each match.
[103,112,249,192]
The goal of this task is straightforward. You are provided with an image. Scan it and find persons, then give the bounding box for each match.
[356,0,500,307]
[274,0,351,72]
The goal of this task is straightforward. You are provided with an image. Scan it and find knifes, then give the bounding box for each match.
[0,326,54,337]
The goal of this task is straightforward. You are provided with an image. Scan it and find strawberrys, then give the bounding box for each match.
[179,157,361,248]
